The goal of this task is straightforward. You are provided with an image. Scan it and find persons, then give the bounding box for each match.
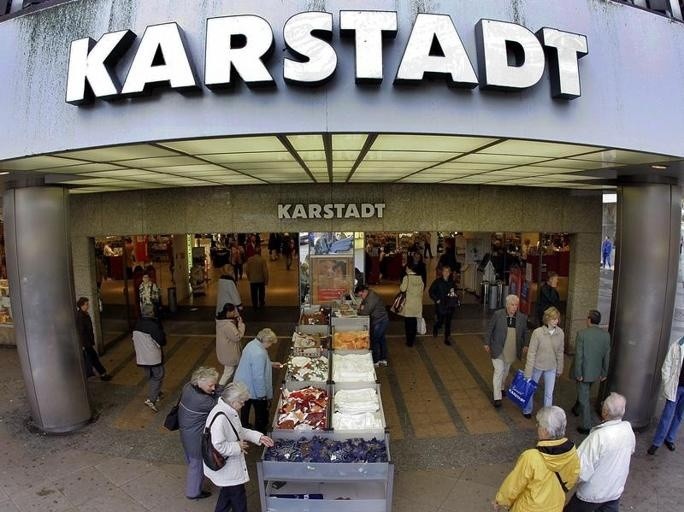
[163,366,223,501]
[232,327,285,432]
[571,390,637,511]
[536,272,560,325]
[482,295,565,418]
[199,380,275,512]
[75,233,299,412]
[493,404,580,511]
[521,239,533,254]
[569,310,612,435]
[601,235,611,267]
[647,335,684,454]
[358,237,460,366]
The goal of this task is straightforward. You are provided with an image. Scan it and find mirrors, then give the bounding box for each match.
[596,189,619,337]
[297,232,365,307]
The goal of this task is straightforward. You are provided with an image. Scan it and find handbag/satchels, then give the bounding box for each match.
[164,406,179,431]
[507,369,538,410]
[202,428,226,470]
[417,318,426,334]
[390,293,405,312]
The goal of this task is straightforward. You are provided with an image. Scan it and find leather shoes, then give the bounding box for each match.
[495,400,501,407]
[501,390,506,396]
[572,408,579,416]
[187,491,210,499]
[648,446,658,454]
[665,440,675,450]
[577,427,589,434]
[524,414,532,419]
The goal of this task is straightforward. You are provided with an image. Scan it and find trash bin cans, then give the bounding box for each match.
[488,284,498,309]
[502,284,510,309]
[167,286,177,313]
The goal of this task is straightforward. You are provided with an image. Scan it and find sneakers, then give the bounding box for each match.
[156,392,164,401]
[144,399,158,412]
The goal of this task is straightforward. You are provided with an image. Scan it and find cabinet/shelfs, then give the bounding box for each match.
[257,427,395,511]
